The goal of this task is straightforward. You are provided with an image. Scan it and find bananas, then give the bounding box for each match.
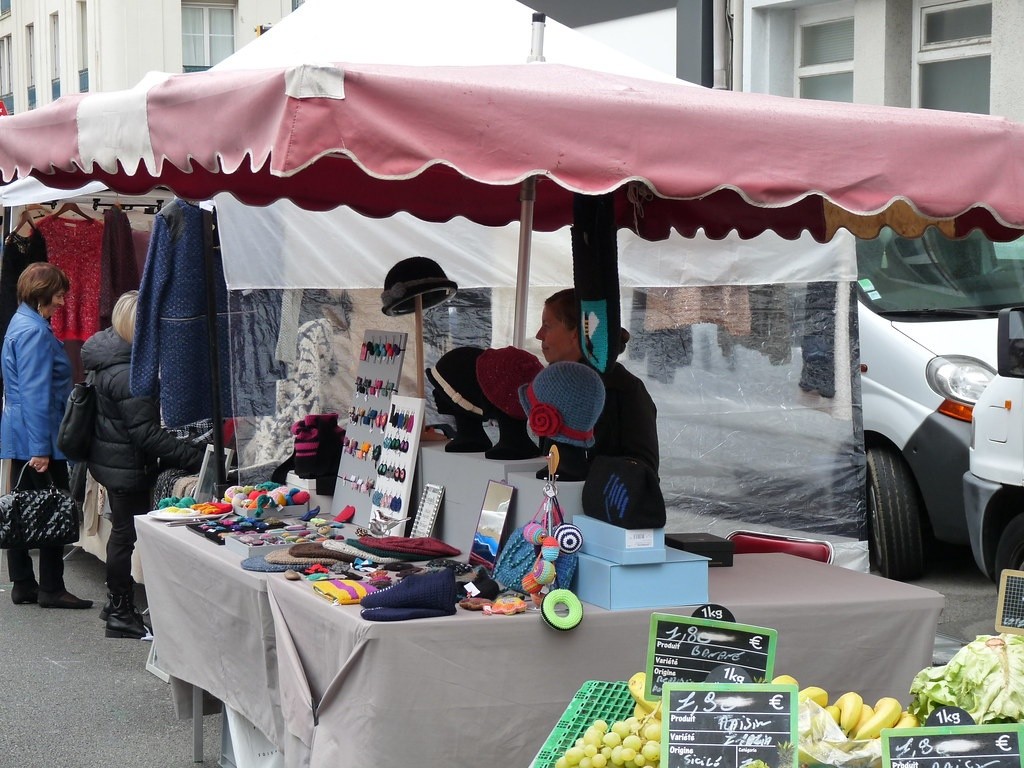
[768,674,921,768]
[628,671,662,722]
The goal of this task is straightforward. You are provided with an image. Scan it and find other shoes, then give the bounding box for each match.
[11,580,38,604]
[38,590,93,608]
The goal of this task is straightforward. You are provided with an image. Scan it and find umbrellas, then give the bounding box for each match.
[0,60,1024,352]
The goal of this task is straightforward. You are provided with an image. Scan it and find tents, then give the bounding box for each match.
[0,0,857,418]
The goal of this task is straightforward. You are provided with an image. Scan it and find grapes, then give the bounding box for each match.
[554,716,663,768]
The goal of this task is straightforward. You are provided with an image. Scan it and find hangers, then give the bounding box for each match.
[6,202,91,243]
[115,191,123,212]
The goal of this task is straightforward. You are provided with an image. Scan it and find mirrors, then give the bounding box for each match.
[144,446,231,682]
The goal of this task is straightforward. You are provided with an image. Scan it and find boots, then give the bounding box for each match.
[99,591,153,638]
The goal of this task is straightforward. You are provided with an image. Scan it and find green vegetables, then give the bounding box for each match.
[907,632,1024,727]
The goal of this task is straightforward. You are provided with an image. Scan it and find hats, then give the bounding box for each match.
[517,361,606,447]
[381,257,458,316]
[477,346,544,419]
[426,347,489,416]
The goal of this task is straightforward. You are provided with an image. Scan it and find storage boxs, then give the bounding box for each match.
[286,471,332,515]
[527,680,639,768]
[420,440,734,609]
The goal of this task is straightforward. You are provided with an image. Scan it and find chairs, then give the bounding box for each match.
[727,530,834,564]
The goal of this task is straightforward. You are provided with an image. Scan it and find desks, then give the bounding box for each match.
[76,470,197,583]
[135,514,944,768]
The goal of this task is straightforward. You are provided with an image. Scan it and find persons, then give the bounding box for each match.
[0,262,93,608]
[80,289,206,638]
[535,287,659,485]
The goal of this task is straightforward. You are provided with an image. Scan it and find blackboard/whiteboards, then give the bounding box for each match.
[644,611,778,701]
[660,683,798,768]
[880,723,1024,768]
[995,569,1024,635]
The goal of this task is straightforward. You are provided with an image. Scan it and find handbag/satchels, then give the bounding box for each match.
[491,524,579,596]
[56,381,97,463]
[581,456,667,529]
[0,461,79,549]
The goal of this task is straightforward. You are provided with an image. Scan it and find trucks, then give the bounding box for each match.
[620,227,1023,584]
